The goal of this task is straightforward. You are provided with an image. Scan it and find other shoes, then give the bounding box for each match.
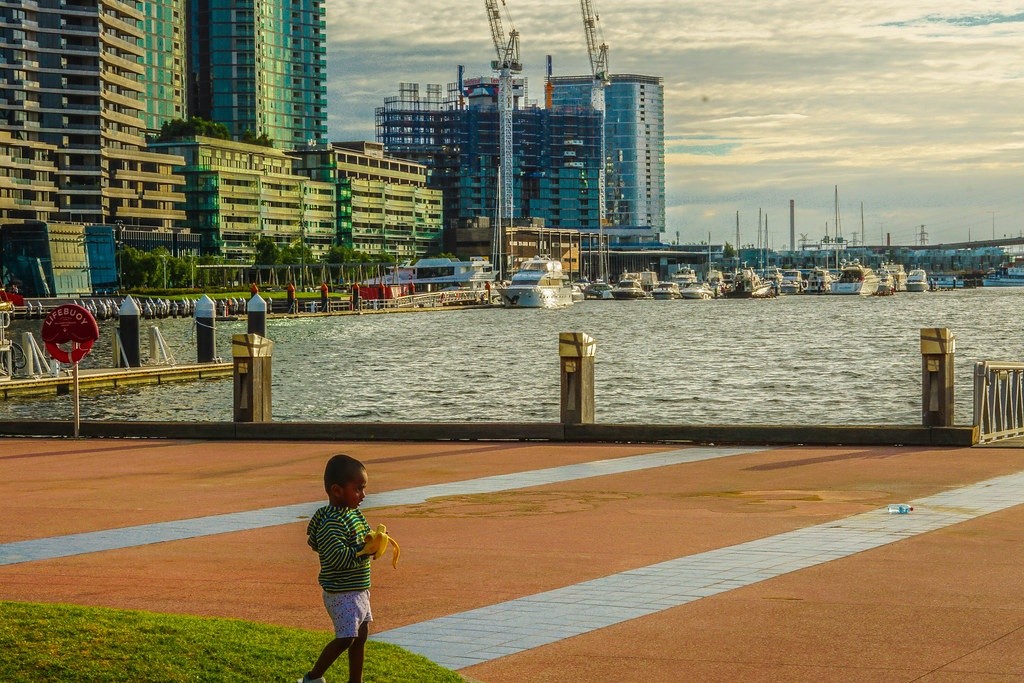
[302,672,326,683]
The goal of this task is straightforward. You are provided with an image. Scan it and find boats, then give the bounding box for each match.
[495,255,573,310]
[581,184,928,301]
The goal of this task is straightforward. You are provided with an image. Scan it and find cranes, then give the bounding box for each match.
[479,0,524,270]
[577,0,612,245]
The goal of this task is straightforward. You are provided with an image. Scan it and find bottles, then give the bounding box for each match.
[888,504,914,513]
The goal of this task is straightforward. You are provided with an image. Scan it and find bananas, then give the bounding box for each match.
[356,523,400,570]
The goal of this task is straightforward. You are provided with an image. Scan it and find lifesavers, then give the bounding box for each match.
[44,337,95,363]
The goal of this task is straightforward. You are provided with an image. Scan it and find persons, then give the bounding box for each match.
[0,283,18,304]
[297,454,389,683]
